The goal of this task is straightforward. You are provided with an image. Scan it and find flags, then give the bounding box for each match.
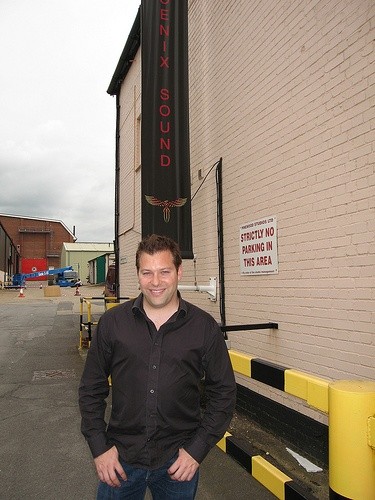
[137,0,197,261]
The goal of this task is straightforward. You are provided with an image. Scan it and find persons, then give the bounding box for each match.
[78,234,239,500]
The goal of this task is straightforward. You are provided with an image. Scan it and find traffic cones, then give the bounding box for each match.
[18,288,25,297]
[74,285,81,296]
